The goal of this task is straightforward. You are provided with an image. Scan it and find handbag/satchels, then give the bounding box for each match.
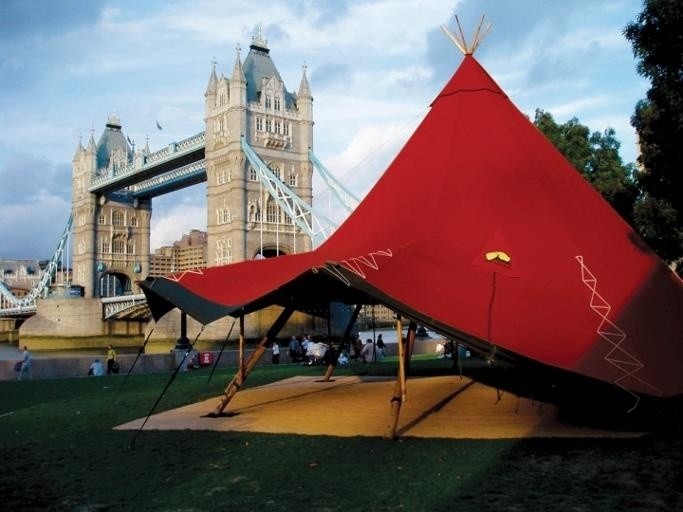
[112,362,119,373]
[14,362,21,372]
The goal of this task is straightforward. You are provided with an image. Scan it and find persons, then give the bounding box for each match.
[88,358,104,377]
[272,340,280,364]
[105,344,119,374]
[402,334,406,351]
[416,325,426,334]
[440,337,452,359]
[16,346,34,382]
[287,333,388,367]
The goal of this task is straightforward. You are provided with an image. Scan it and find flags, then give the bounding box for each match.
[127,135,132,145]
